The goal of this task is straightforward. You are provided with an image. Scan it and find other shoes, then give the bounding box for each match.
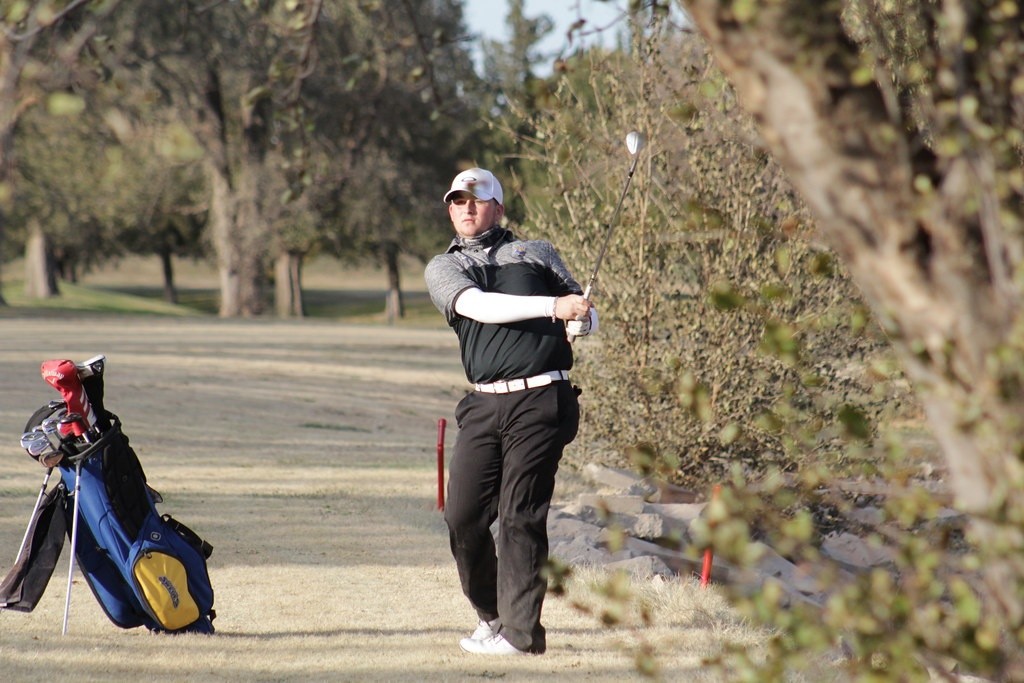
[460,634,536,656]
[471,617,502,640]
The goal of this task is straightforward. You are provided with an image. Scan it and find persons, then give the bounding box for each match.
[423,167,599,659]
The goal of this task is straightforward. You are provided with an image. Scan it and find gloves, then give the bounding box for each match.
[566,320,590,336]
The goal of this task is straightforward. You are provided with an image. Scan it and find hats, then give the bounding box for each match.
[443,167,503,206]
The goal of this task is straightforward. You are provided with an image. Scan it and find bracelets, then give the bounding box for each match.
[552,295,559,324]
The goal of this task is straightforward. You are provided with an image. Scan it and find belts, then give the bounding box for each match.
[475,370,569,394]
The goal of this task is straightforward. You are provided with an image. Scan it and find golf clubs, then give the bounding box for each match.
[21,399,102,467]
[567,132,644,343]
